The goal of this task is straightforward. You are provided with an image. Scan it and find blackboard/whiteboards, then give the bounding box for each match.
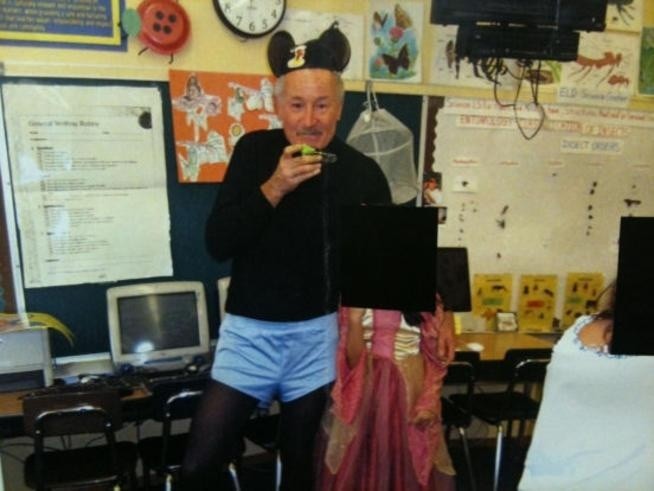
[0,74,430,364]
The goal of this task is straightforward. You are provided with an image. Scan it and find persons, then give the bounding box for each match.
[425,177,442,203]
[516,278,653,491]
[315,294,461,489]
[175,68,395,489]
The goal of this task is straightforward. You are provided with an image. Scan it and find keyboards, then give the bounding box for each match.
[141,370,208,396]
[18,378,133,399]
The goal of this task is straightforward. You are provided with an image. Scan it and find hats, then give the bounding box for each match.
[267,21,351,78]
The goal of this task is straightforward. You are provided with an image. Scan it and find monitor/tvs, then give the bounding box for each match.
[107,281,209,374]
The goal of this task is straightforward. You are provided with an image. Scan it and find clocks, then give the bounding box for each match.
[213,0,286,40]
